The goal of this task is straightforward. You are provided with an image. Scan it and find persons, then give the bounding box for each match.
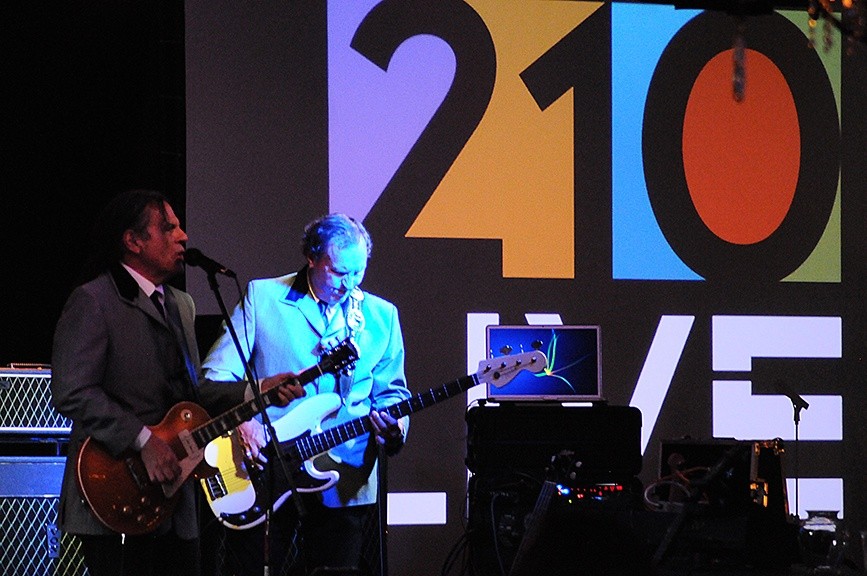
[50,190,306,576]
[201,213,411,576]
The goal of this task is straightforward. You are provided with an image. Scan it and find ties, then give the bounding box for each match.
[318,300,329,328]
[151,291,165,321]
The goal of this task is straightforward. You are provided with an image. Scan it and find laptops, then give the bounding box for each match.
[485,324,603,406]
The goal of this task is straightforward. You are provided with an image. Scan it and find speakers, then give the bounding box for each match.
[464,406,644,576]
[0,368,92,576]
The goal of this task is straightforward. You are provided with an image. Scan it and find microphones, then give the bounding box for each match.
[773,381,809,410]
[183,248,236,278]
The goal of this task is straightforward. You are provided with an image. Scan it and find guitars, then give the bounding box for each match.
[197,338,548,530]
[76,337,359,535]
[510,451,582,576]
